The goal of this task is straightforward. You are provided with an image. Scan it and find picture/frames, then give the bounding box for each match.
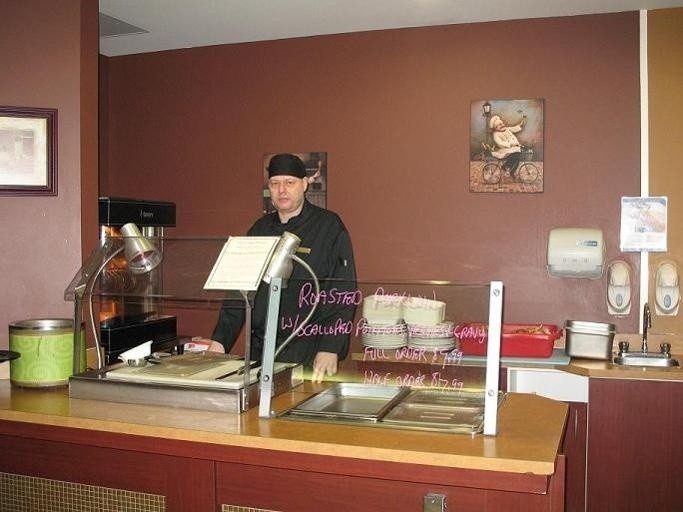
[0,106,59,197]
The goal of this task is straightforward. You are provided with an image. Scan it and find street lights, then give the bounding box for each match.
[482,103,491,143]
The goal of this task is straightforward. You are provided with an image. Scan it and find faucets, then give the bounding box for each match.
[642,303,652,352]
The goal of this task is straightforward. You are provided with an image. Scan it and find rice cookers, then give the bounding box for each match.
[7,317,85,390]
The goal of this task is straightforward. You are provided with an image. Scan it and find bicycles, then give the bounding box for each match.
[483,145,538,183]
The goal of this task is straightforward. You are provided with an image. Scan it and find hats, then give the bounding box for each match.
[268,153,306,179]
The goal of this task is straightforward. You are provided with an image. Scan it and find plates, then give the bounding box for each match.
[359,292,456,352]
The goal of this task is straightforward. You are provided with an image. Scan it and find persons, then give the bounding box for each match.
[488,112,528,180]
[202,152,358,387]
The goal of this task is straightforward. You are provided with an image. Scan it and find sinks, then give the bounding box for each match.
[615,354,676,369]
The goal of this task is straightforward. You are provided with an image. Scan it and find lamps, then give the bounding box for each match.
[88,221,163,371]
